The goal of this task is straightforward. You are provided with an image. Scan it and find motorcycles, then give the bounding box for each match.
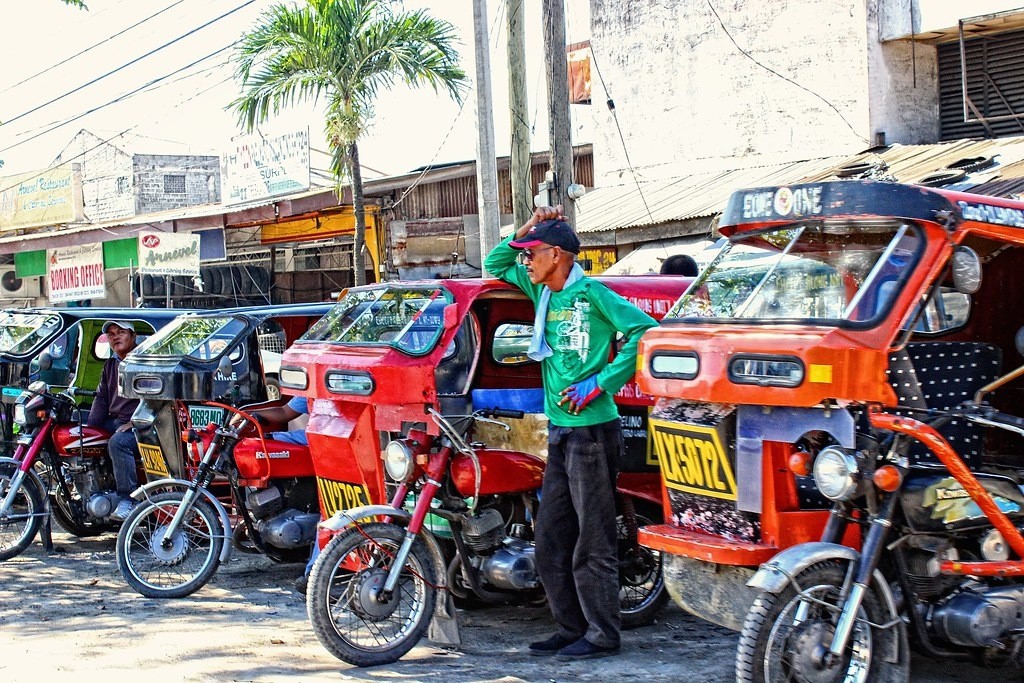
[733,329,1024,683]
[0,349,126,563]
[114,354,321,599]
[306,403,672,668]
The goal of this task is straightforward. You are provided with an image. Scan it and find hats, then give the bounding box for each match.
[102,319,137,335]
[508,218,581,256]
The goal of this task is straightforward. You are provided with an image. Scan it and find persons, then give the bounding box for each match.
[660,255,698,277]
[483,204,660,661]
[72,322,138,522]
[228,396,315,445]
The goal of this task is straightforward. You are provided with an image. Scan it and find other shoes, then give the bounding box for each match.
[294,577,308,595]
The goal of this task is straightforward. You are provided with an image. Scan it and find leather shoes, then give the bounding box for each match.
[527,632,624,662]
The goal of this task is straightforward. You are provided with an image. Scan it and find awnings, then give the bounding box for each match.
[225,204,384,285]
[499,137,1024,248]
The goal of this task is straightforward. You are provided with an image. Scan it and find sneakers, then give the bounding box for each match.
[112,500,137,521]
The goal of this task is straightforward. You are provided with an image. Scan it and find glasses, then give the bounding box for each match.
[518,246,556,261]
